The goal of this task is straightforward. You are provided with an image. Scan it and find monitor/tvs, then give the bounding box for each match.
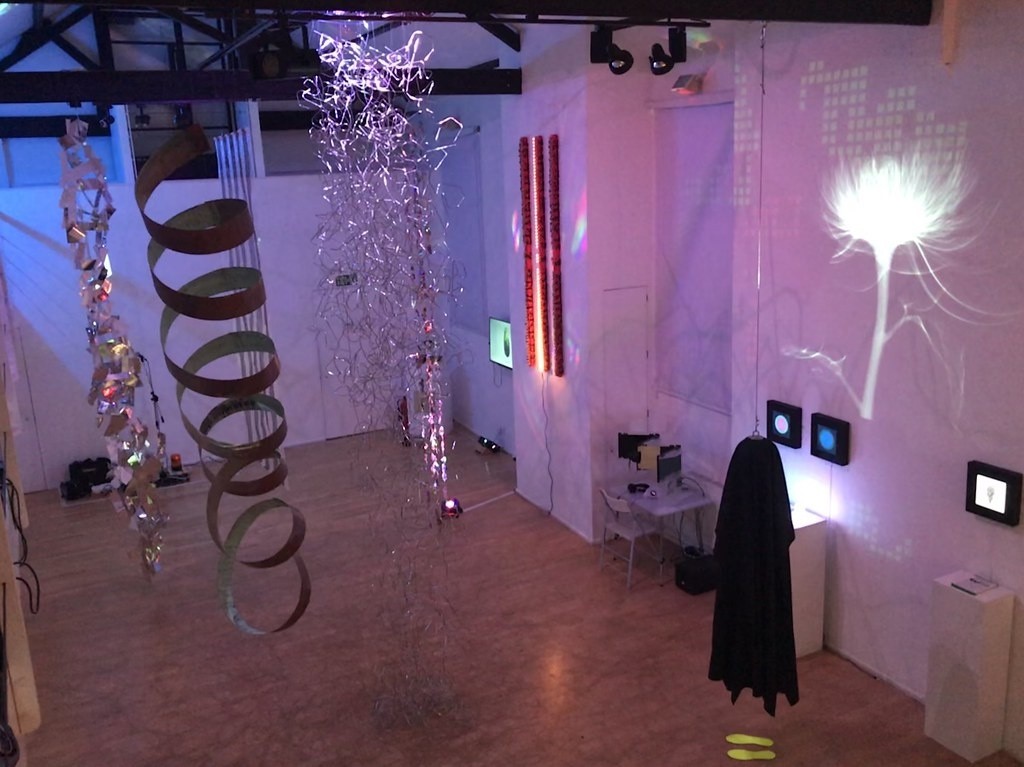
[656,444,681,490]
[488,315,516,372]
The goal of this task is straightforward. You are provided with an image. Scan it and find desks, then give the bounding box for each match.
[612,482,711,587]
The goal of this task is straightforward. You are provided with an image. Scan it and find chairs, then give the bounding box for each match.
[597,489,663,592]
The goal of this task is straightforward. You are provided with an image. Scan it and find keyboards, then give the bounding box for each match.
[643,476,673,498]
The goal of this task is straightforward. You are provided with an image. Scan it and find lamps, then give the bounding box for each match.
[604,44,634,76]
[648,43,675,75]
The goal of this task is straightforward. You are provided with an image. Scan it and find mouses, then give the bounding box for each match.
[637,483,649,491]
